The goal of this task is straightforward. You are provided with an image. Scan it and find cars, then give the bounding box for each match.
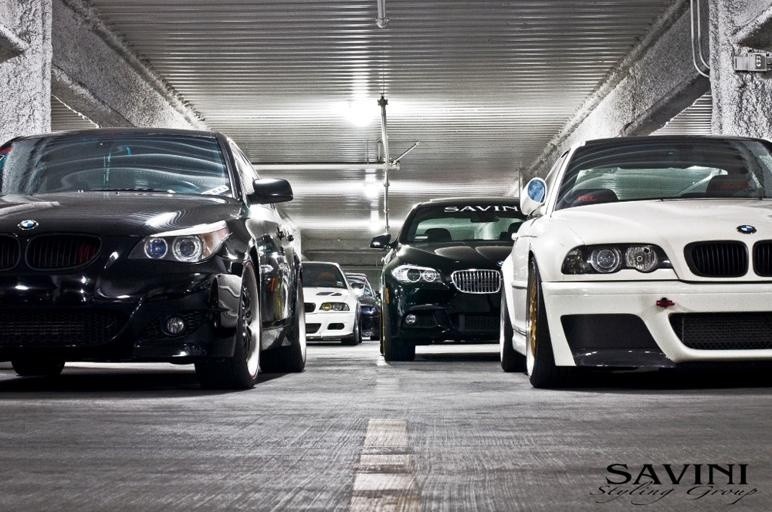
[497,135,772,389]
[301,260,382,348]
[0,127,308,392]
[370,196,529,362]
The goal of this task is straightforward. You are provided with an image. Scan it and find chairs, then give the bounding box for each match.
[557,188,618,211]
[499,221,523,241]
[705,173,756,198]
[422,229,452,244]
